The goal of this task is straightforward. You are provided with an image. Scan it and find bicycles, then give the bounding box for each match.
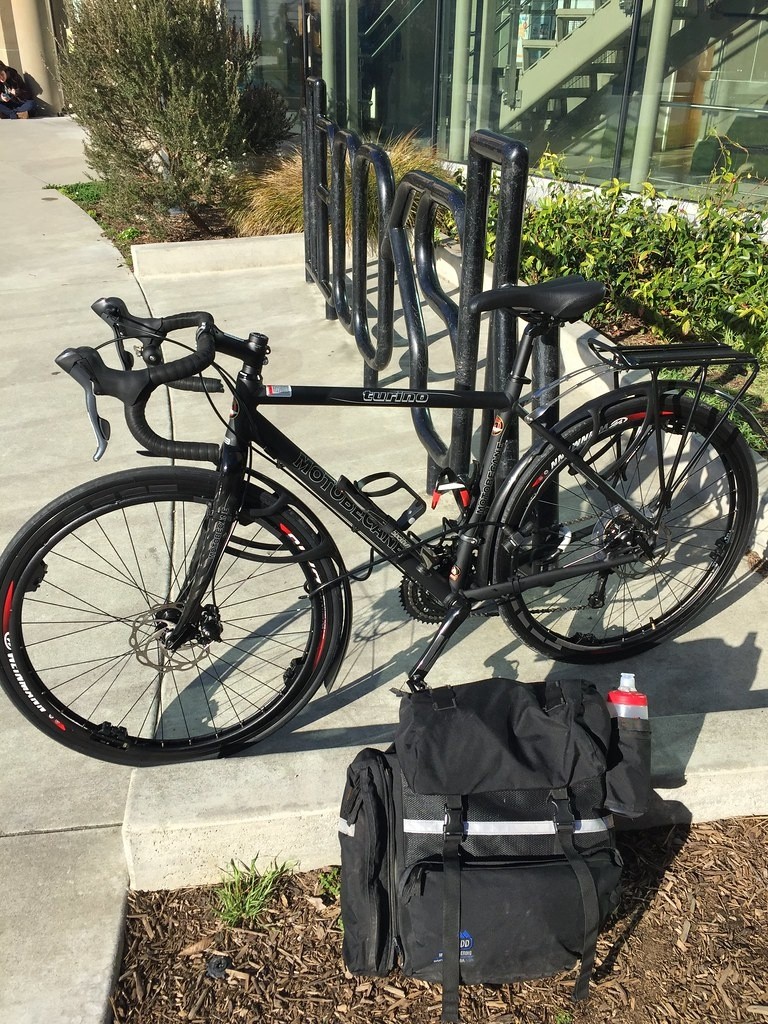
[0,275,768,767]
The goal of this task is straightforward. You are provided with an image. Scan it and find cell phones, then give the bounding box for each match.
[3,94,11,100]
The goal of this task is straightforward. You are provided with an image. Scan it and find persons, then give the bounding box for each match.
[0,61,39,119]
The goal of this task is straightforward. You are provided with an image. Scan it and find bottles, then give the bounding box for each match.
[606,672,649,720]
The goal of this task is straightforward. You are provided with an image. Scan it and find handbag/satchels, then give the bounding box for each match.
[338,680,652,1022]
